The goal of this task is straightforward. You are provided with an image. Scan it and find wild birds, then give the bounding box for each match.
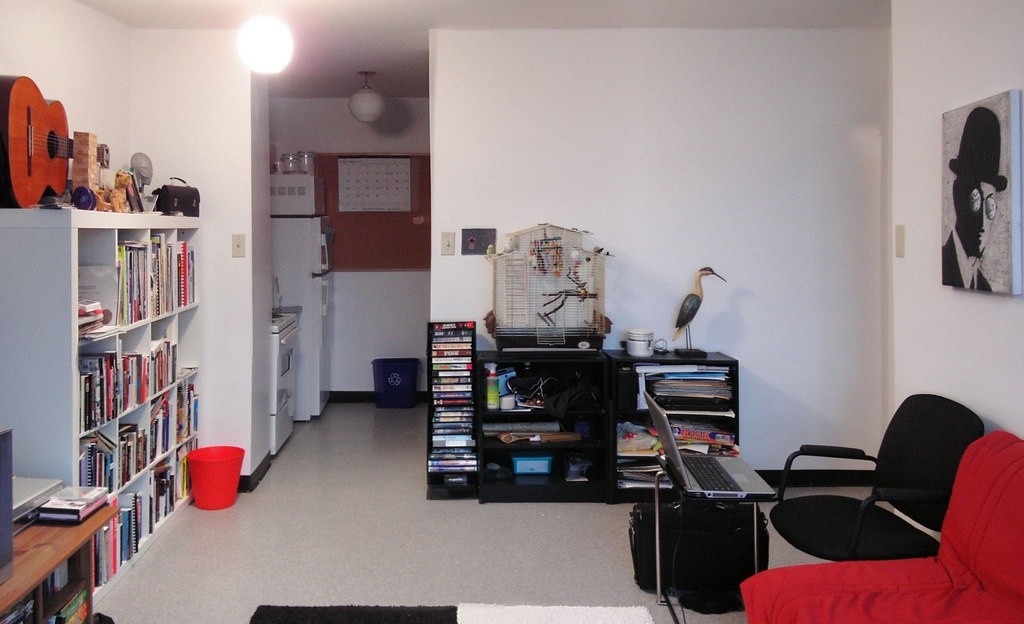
[672,266,728,350]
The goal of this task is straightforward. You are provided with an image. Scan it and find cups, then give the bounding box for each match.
[273,151,314,176]
[139,192,159,212]
[626,328,653,358]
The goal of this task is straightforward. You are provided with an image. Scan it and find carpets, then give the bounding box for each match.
[250,603,655,624]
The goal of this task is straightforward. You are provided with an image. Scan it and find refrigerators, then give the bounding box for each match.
[270,214,335,422]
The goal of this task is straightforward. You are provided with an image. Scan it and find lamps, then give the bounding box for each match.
[350,72,385,121]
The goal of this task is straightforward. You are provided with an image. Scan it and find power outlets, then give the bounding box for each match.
[462,229,496,254]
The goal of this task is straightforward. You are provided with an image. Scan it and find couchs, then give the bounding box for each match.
[739,431,1024,624]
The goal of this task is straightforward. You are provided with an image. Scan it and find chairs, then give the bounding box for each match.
[770,394,984,561]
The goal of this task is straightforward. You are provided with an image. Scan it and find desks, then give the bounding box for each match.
[655,453,778,604]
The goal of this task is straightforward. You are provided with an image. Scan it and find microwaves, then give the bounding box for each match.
[269,174,326,216]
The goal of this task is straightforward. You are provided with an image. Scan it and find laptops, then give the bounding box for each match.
[643,390,776,499]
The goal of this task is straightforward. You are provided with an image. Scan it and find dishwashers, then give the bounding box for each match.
[271,314,300,458]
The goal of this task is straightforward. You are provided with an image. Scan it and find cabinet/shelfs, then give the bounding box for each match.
[426,321,478,499]
[0,507,120,624]
[479,351,739,504]
[0,209,200,607]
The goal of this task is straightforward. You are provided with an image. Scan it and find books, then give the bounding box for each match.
[80,341,199,587]
[0,558,90,624]
[653,366,732,400]
[39,485,108,523]
[428,324,478,485]
[78,233,195,339]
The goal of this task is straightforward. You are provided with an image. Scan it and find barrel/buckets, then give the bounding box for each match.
[187,446,245,509]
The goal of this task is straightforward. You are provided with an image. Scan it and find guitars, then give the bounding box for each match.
[0,74,111,209]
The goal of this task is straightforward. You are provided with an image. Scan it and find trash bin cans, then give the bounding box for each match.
[371,357,421,410]
